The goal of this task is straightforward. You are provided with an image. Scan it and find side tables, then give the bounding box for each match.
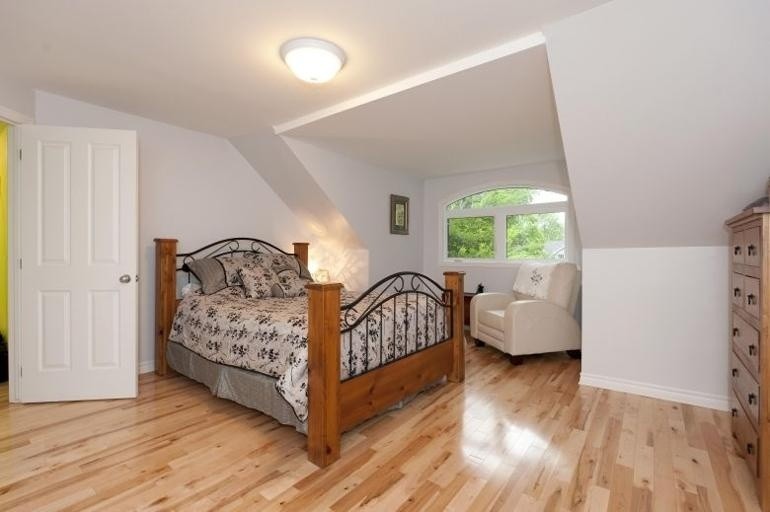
[441,291,476,326]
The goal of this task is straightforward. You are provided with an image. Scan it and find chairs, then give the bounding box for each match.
[469,260,581,365]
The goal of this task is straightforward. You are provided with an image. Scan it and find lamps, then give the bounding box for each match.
[279,37,345,84]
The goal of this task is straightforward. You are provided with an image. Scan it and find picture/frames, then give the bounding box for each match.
[390,194,410,235]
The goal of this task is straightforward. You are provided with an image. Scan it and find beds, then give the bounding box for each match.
[154,237,467,468]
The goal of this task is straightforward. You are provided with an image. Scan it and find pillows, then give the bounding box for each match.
[185,254,313,298]
[512,261,578,309]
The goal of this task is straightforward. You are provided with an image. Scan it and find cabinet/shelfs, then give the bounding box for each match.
[723,206,770,512]
